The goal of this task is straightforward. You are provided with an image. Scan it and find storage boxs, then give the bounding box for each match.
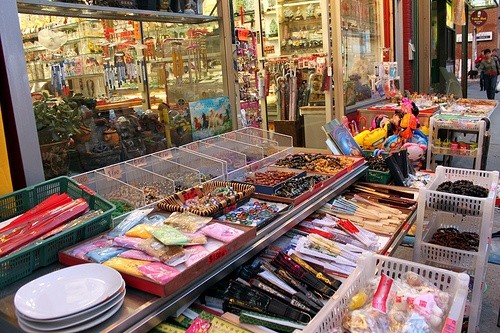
[0,175,116,289]
[413,165,500,333]
[301,250,470,333]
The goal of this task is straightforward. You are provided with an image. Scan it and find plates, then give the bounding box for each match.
[14,263,126,333]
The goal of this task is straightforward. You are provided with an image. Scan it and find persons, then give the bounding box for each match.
[477,49,500,100]
[269,19,279,37]
[475,50,486,91]
[281,4,322,21]
[491,49,500,93]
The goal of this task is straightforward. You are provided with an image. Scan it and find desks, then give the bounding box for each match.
[299,105,336,149]
[94,98,162,112]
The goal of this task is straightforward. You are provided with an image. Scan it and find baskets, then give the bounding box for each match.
[422,165,500,272]
[291,250,470,333]
[0,176,116,289]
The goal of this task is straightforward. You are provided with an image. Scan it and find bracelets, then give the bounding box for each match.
[435,179,489,198]
[428,225,479,252]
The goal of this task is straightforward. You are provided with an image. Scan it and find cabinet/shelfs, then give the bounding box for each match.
[356,104,433,133]
[0,146,419,333]
[425,98,498,173]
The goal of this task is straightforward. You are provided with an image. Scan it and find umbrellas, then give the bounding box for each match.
[301,70,314,107]
[273,61,303,121]
[258,66,270,98]
[349,116,367,136]
